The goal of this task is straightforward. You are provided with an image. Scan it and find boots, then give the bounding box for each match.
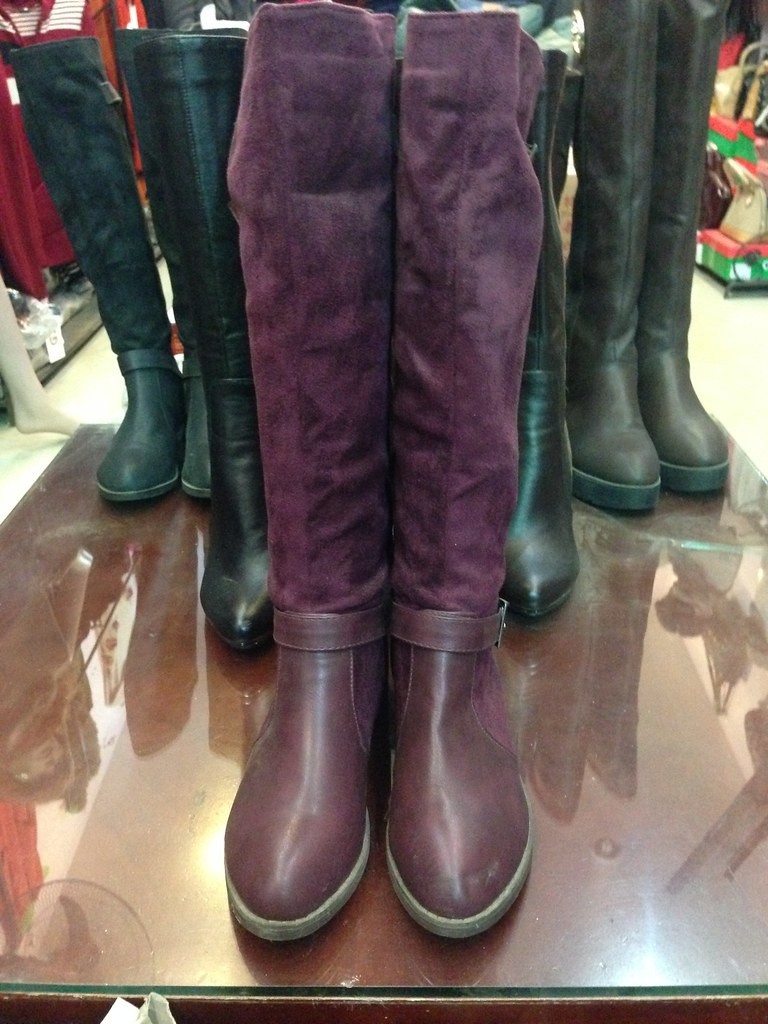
[638,0,730,509]
[8,28,248,501]
[225,3,396,940]
[382,11,541,938]
[132,35,274,648]
[502,49,582,618]
[566,0,661,510]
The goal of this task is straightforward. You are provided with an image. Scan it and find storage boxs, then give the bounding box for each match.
[698,228,768,283]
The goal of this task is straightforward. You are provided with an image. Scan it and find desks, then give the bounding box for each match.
[0,421,764,1021]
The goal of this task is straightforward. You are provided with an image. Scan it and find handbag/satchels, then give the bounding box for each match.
[700,143,733,228]
[714,41,768,136]
[701,624,745,713]
[720,158,768,244]
